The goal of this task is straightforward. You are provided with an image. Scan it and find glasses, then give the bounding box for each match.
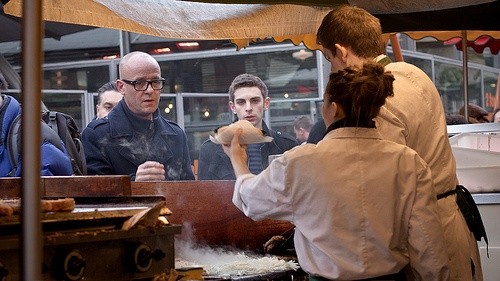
[121,77,166,91]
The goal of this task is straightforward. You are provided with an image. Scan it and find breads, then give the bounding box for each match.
[0,198,76,216]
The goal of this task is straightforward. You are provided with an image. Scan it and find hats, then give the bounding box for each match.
[459,103,489,117]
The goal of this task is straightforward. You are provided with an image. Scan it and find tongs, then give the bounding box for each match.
[277,225,295,252]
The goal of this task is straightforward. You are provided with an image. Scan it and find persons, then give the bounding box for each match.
[196,73,301,181]
[1,92,75,175]
[315,3,491,281]
[79,50,198,181]
[443,102,500,126]
[293,116,314,145]
[220,61,453,281]
[95,79,129,120]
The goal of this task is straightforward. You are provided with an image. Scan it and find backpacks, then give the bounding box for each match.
[8,101,87,177]
